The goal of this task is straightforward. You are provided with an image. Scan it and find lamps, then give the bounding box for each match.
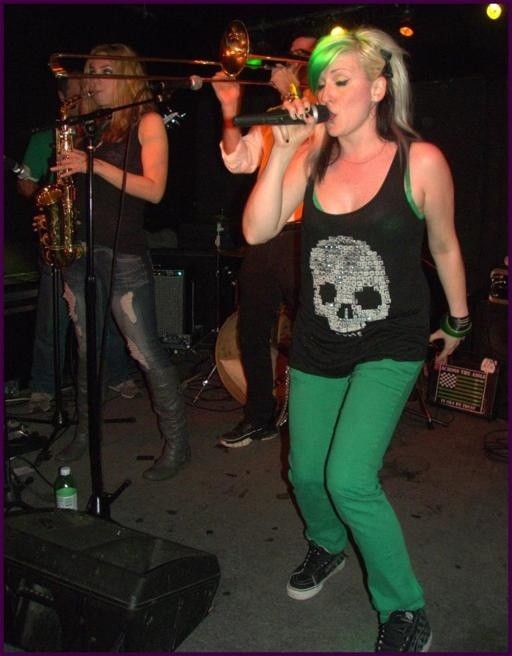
[396,2,508,39]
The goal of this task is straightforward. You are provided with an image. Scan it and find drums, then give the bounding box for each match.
[215,304,296,410]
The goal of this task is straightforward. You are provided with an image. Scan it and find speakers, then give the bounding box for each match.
[0,507,221,656]
[477,300,512,420]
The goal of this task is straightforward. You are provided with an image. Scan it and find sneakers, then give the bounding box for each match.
[285,540,347,601]
[122,379,139,399]
[219,417,279,448]
[28,391,54,413]
[375,609,433,652]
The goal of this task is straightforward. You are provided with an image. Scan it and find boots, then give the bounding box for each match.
[55,376,105,463]
[142,366,192,482]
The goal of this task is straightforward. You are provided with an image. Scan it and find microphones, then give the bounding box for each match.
[233,103,329,126]
[150,75,202,91]
[4,155,31,181]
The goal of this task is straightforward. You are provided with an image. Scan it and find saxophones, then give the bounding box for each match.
[34,90,103,265]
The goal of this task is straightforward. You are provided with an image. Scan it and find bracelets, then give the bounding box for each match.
[441,314,473,337]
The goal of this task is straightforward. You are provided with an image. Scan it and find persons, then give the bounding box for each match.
[242,24,471,654]
[16,68,147,412]
[214,38,321,449]
[49,42,192,482]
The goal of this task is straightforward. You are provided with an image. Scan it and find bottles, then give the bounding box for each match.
[53,466,81,510]
[9,160,41,189]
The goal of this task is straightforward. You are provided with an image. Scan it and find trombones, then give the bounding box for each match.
[47,19,309,88]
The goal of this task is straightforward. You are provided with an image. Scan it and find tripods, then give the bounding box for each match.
[79,124,154,537]
[0,265,135,467]
[181,216,224,406]
